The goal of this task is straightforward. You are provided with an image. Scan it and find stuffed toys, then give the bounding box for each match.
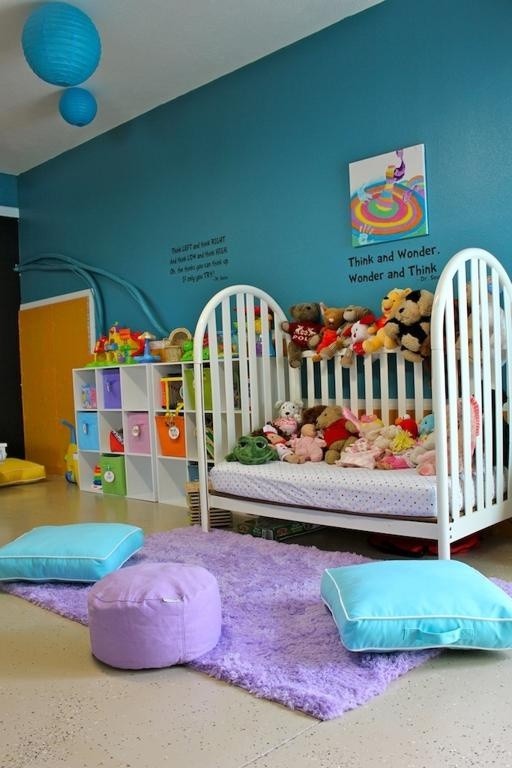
[226,396,481,475]
[280,288,476,371]
[226,286,477,477]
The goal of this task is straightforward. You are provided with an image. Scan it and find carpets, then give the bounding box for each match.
[0,526,512,720]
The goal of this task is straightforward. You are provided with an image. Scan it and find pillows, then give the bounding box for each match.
[322,558,512,653]
[0,458,47,486]
[0,523,144,583]
[89,563,221,669]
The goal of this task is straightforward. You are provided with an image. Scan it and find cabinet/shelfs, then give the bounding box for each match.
[72,356,289,508]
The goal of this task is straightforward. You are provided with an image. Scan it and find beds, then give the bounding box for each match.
[194,246,512,558]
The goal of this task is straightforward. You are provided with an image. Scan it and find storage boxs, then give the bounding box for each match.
[103,369,121,409]
[155,415,186,457]
[188,465,212,482]
[99,455,126,496]
[184,369,212,410]
[77,411,99,450]
[127,413,150,454]
